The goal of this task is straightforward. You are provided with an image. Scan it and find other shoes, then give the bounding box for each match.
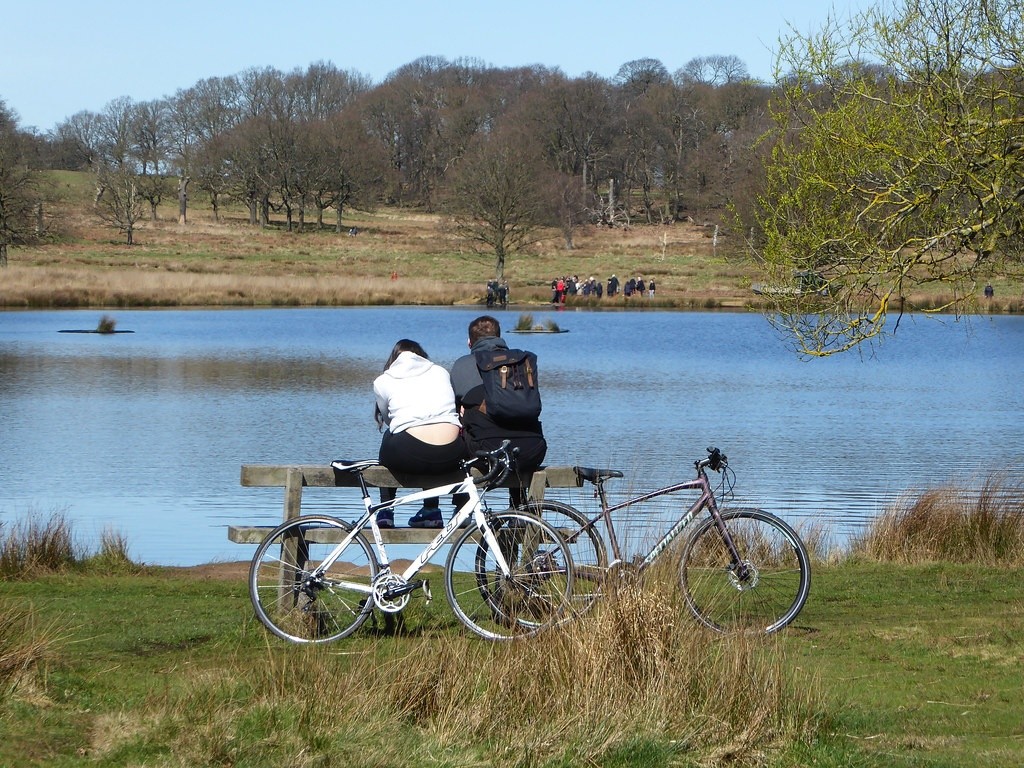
[448,509,471,528]
[509,519,526,527]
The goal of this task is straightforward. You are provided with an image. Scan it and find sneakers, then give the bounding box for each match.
[408,507,443,528]
[376,509,394,527]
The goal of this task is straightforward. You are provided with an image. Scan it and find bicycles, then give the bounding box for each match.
[473,447,813,638]
[247,438,575,646]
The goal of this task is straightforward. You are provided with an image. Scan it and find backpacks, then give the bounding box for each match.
[471,350,542,421]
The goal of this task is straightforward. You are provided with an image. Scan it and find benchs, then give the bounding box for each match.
[229,464,585,614]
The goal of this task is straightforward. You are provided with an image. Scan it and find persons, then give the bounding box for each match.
[636,277,645,296]
[486,277,509,305]
[649,279,655,297]
[596,281,602,298]
[624,281,632,297]
[630,277,636,294]
[450,316,547,528]
[590,277,597,296]
[550,275,578,304]
[583,279,590,295]
[373,339,462,528]
[576,280,584,296]
[607,274,619,296]
